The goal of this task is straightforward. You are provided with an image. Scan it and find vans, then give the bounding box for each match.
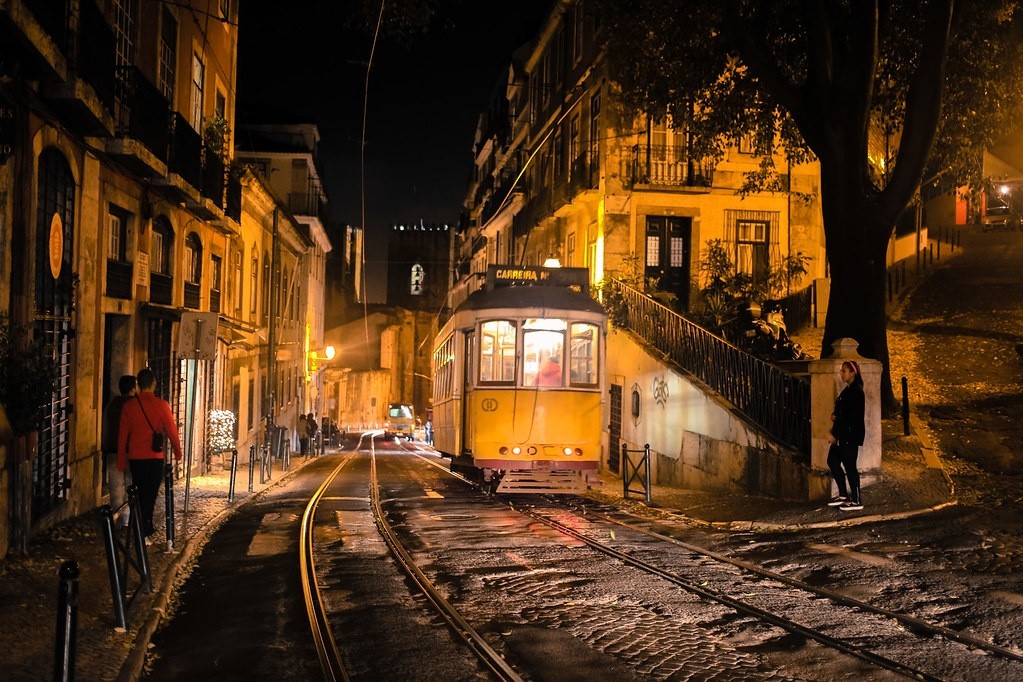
[384,403,415,442]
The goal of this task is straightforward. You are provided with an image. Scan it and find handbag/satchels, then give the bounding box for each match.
[152,432,163,452]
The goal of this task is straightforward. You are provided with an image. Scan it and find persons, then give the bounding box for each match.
[297,413,318,456]
[425,420,434,445]
[530,356,562,386]
[116,368,183,546]
[826,360,866,511]
[104,374,137,528]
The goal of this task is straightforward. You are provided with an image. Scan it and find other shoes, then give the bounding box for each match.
[828,496,850,506]
[838,501,863,510]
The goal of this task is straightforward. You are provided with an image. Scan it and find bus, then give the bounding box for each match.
[430,264,608,494]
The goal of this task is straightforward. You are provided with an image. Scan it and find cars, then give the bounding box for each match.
[982,207,1016,232]
[322,417,339,441]
